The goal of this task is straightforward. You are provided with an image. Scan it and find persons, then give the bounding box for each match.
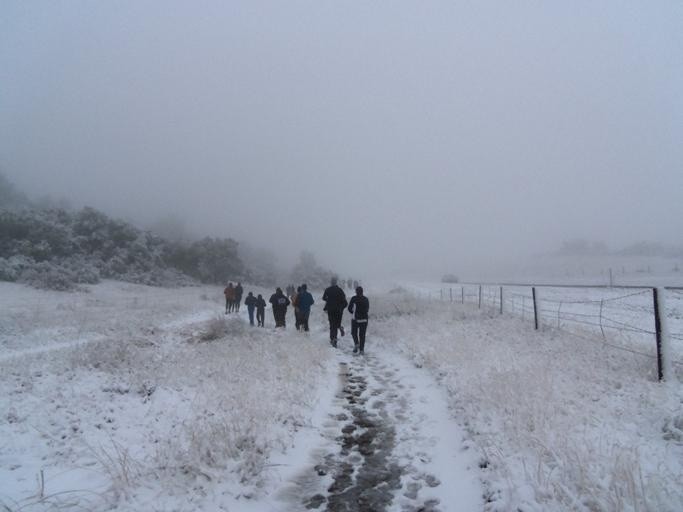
[223,275,371,356]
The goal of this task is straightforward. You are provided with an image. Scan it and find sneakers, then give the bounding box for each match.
[353,344,364,355]
[330,339,337,347]
